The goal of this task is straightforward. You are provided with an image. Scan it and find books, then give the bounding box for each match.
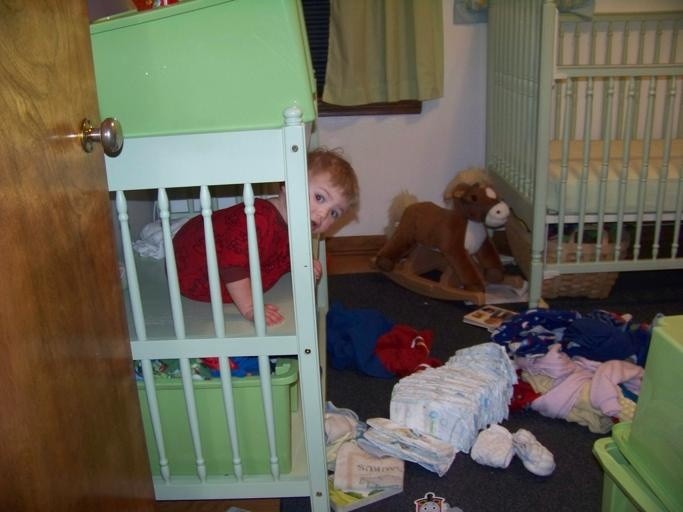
[461,303,518,331]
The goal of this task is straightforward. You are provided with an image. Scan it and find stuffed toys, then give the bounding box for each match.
[368,168,525,306]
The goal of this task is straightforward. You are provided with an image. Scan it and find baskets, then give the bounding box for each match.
[504,210,631,299]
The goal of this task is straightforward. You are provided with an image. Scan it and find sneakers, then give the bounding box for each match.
[470,424,516,468]
[513,428,556,476]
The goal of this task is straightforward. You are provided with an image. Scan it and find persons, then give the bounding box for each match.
[164,146,360,326]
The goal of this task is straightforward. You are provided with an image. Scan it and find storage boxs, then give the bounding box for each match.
[610,316,683,512]
[90,0,317,137]
[592,436,683,512]
[136,360,298,476]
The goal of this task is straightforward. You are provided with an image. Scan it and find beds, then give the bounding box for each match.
[104,104,330,512]
[487,0,682,314]
